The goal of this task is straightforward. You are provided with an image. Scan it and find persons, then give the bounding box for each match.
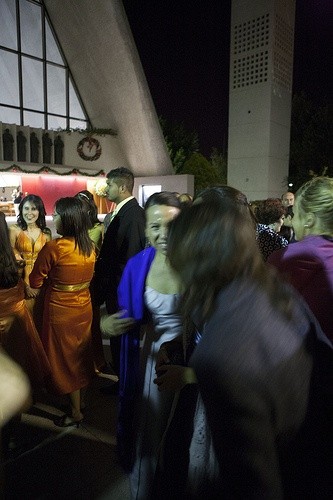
[0,167,333,429]
[166,199,333,500]
[101,191,193,500]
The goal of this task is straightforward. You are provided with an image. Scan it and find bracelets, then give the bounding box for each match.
[25,285,30,290]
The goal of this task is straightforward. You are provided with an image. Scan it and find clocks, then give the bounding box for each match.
[77,137,102,161]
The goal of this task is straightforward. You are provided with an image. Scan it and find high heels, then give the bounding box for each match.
[54,413,84,428]
